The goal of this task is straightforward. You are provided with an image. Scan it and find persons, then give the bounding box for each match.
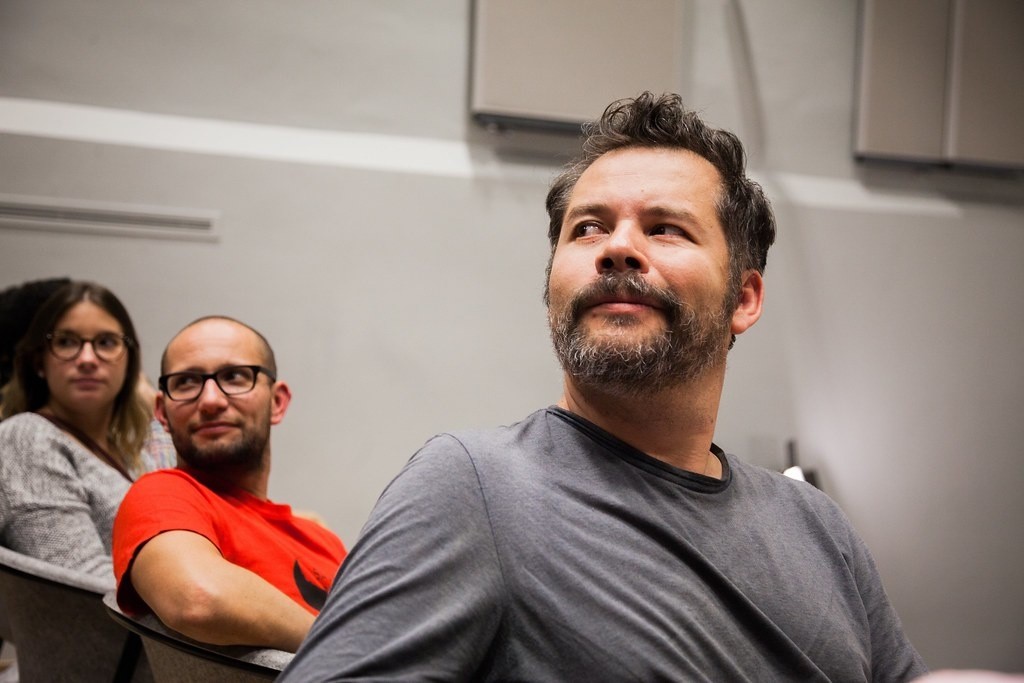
[0,277,181,592]
[277,90,932,683]
[111,317,349,656]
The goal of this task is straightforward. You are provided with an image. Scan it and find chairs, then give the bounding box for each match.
[101,591,297,682]
[0,545,152,682]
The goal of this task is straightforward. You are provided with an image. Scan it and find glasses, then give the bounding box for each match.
[157,366,278,403]
[43,328,132,363]
